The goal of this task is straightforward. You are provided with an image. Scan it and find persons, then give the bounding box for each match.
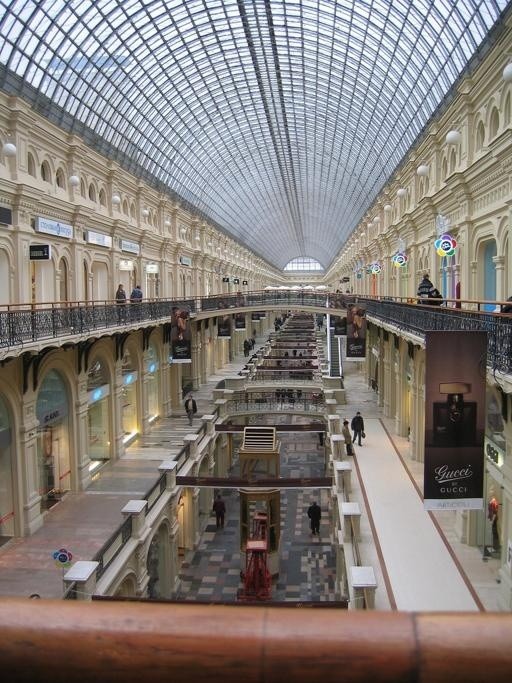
[487,494,503,550]
[183,394,197,425]
[500,297,512,313]
[115,284,127,319]
[350,410,364,447]
[129,285,142,323]
[235,288,379,404]
[416,274,437,306]
[431,288,443,306]
[342,420,355,457]
[212,494,226,529]
[319,432,324,446]
[433,393,475,442]
[171,306,188,341]
[307,500,321,534]
